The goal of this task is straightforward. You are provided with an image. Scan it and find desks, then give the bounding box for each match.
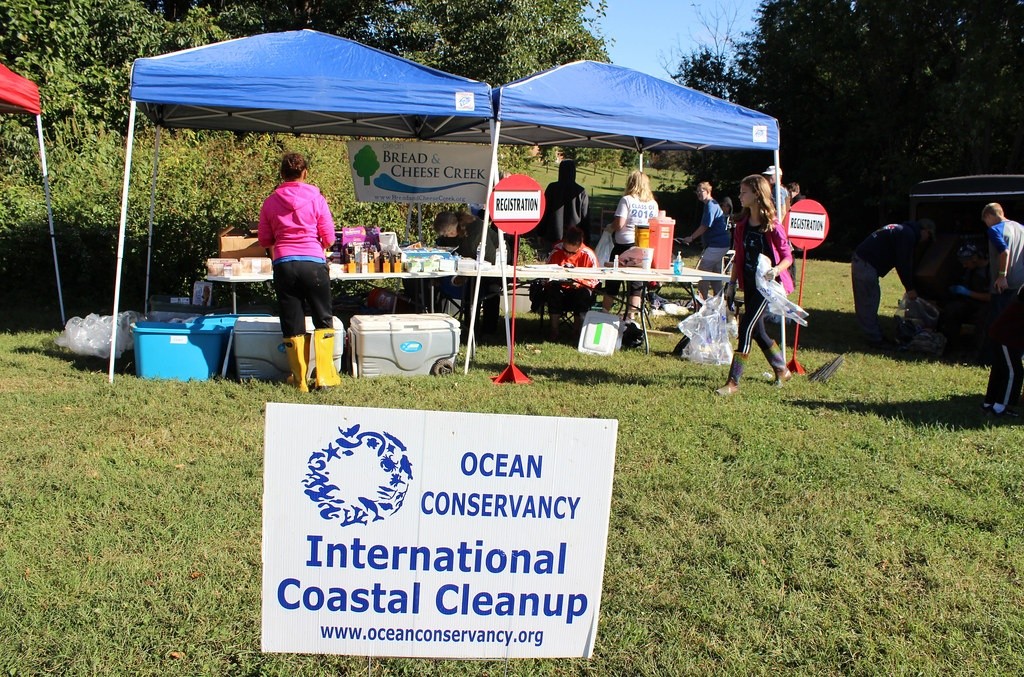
[206,265,731,379]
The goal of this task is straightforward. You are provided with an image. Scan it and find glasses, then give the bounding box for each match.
[562,242,581,255]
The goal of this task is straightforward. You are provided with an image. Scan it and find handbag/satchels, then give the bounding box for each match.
[594,231,615,267]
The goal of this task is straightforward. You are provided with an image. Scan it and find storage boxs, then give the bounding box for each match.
[149,294,217,314]
[403,258,491,270]
[207,226,272,277]
[580,313,625,349]
[129,313,460,383]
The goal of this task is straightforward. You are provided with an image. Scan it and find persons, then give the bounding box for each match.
[713,174,793,395]
[259,154,341,391]
[435,158,801,343]
[853,217,937,350]
[935,202,1024,420]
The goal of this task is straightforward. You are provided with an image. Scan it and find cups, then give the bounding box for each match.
[641,247,655,269]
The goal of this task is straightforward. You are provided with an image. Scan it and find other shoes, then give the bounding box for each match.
[992,405,1019,417]
[870,337,896,352]
[984,404,994,412]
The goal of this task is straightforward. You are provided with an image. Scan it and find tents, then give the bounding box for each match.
[0,64,67,325]
[106,30,516,384]
[463,58,788,378]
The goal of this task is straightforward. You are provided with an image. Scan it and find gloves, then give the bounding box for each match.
[954,286,972,296]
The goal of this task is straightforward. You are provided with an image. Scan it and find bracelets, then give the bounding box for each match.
[999,272,1007,277]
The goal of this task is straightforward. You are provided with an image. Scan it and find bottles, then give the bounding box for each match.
[614,255,619,272]
[477,241,483,270]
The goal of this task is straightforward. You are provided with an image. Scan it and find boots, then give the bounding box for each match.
[314,327,341,393]
[283,334,309,393]
[763,340,793,388]
[714,351,749,397]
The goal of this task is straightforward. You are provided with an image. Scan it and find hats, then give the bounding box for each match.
[917,218,936,243]
[762,166,783,176]
